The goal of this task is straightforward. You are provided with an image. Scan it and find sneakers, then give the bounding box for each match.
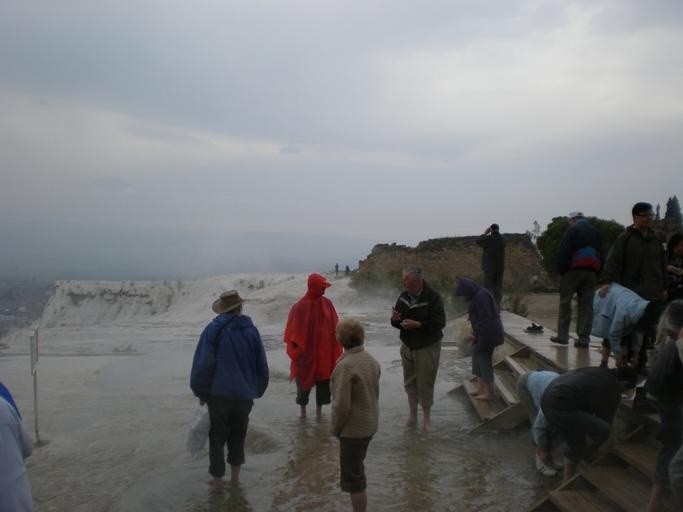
[535,456,558,477]
[550,336,569,345]
[574,341,588,348]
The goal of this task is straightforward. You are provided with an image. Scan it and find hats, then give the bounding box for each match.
[569,210,583,220]
[212,290,249,314]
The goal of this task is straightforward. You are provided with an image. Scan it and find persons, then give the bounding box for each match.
[190,290,269,494]
[517,202,683,512]
[284,273,343,421]
[328,318,381,512]
[455,277,504,401]
[0,380,35,512]
[476,223,506,313]
[390,266,446,434]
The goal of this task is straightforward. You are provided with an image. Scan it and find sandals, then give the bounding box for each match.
[524,323,544,333]
